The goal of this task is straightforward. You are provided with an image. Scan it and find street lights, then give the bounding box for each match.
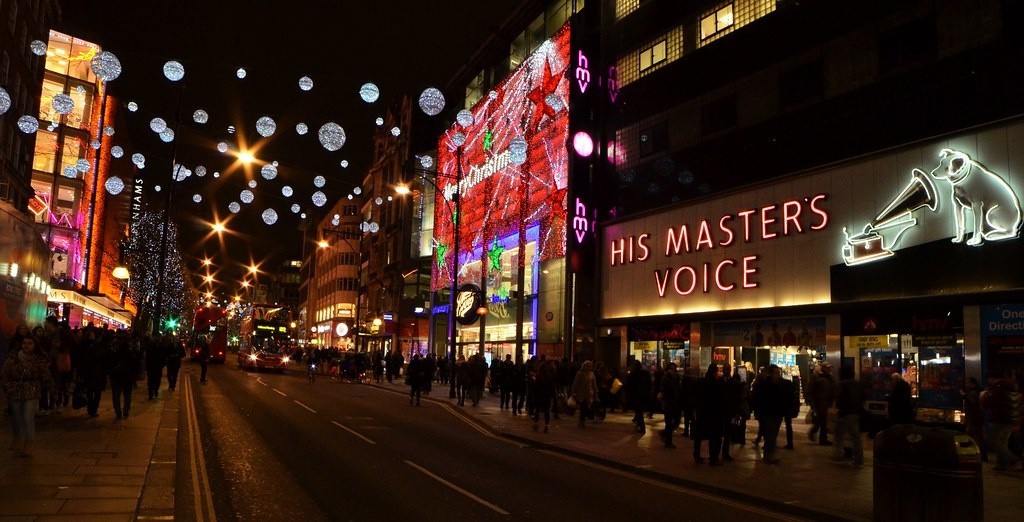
[395,145,488,398]
[318,222,384,352]
[112,110,256,335]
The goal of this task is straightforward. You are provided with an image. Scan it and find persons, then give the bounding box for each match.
[0,305,210,458]
[293,334,1023,475]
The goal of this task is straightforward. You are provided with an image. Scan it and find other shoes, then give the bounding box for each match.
[12,440,38,456]
[86,405,99,417]
[577,420,587,428]
[200,377,208,384]
[553,410,562,420]
[609,407,616,413]
[48,396,56,409]
[471,399,481,406]
[148,387,159,400]
[532,422,548,434]
[421,391,430,396]
[408,395,422,407]
[763,448,779,456]
[805,431,833,445]
[457,397,465,406]
[723,450,734,460]
[512,407,522,416]
[991,453,1018,474]
[490,389,500,394]
[680,431,690,436]
[828,453,867,469]
[632,418,646,433]
[56,404,65,416]
[647,412,654,419]
[620,407,629,414]
[500,401,509,409]
[167,380,177,390]
[660,429,676,448]
[694,453,722,468]
[372,370,449,388]
[528,409,536,417]
[783,441,793,449]
[752,439,759,447]
[113,407,130,418]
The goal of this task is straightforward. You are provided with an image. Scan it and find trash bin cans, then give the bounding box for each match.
[871,422,985,522]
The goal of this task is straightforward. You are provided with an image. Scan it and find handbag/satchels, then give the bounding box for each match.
[804,408,816,424]
[58,351,70,373]
[597,404,606,420]
[729,423,744,443]
[690,422,710,440]
[72,397,87,409]
[567,396,577,408]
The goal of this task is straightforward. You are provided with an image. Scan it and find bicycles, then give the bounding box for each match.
[307,365,372,386]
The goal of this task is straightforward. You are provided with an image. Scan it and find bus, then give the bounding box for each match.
[237,302,294,372]
[190,307,229,363]
